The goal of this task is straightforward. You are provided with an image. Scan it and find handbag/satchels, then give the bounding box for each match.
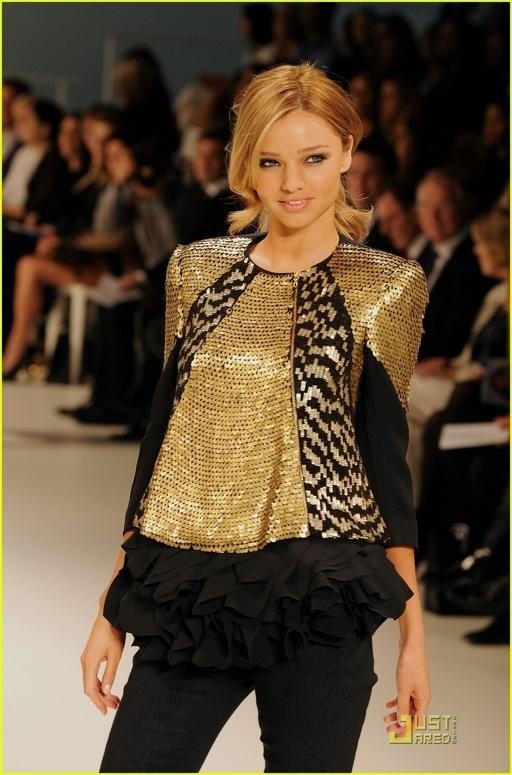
[425,523,509,616]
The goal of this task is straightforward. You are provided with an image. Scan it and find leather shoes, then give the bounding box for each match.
[465,622,509,645]
[58,401,146,441]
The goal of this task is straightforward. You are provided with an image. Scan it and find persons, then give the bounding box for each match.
[77,58,433,774]
[4,1,510,648]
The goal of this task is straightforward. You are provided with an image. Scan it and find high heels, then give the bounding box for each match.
[2,348,32,381]
